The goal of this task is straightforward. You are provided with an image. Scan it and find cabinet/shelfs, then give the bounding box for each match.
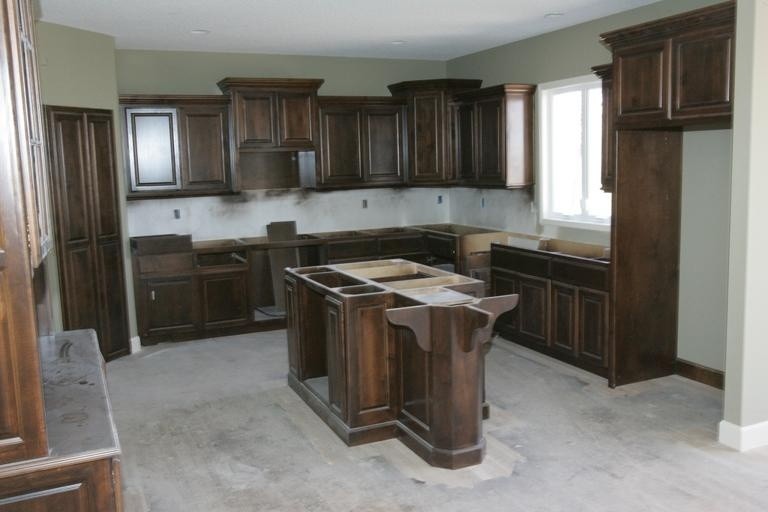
[44,103,131,362]
[314,230,378,264]
[239,234,326,335]
[361,226,427,268]
[132,235,253,348]
[552,244,679,390]
[279,258,520,469]
[0,0,56,466]
[120,97,231,199]
[317,95,406,191]
[406,222,508,337]
[388,79,448,190]
[448,82,536,193]
[598,0,736,131]
[219,79,325,153]
[486,237,577,357]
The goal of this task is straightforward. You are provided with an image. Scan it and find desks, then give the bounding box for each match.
[0,327,124,512]
